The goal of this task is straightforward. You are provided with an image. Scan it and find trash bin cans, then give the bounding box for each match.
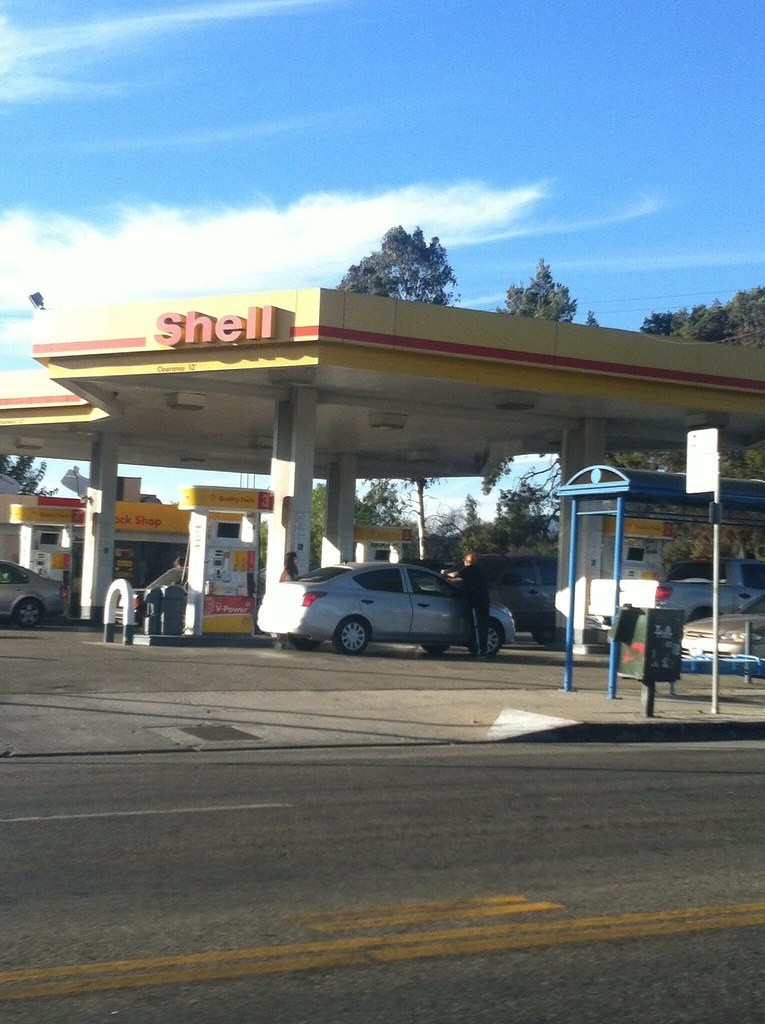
[143,587,187,634]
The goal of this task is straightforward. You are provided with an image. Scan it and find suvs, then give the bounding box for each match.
[440,552,558,644]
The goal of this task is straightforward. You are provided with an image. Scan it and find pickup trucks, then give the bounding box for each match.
[589,557,765,631]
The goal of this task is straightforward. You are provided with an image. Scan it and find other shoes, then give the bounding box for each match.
[467,654,479,659]
[479,653,487,659]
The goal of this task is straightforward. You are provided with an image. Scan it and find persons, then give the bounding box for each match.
[174,557,185,567]
[440,553,491,660]
[270,552,299,649]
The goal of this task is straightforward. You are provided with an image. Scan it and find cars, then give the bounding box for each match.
[131,567,265,622]
[0,559,67,629]
[257,562,516,656]
[675,587,765,664]
